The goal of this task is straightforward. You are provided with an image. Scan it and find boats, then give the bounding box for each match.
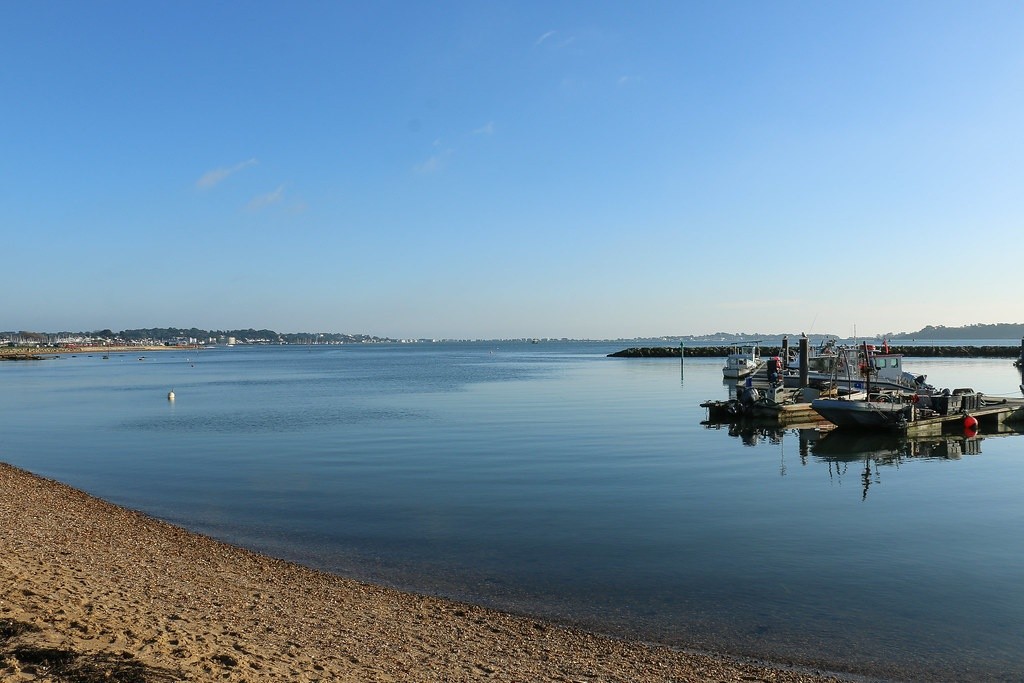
[783,339,935,395]
[722,354,757,378]
[809,341,912,429]
